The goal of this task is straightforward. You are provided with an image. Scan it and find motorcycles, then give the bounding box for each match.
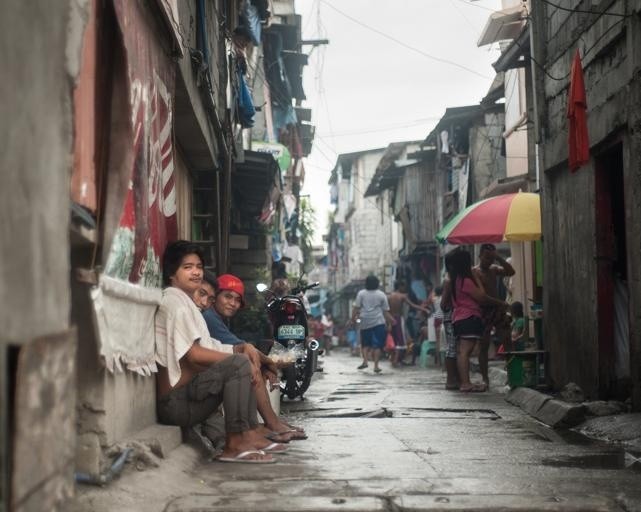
[257,263,322,400]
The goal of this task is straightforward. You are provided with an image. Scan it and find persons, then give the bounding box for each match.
[191,269,307,445]
[202,272,308,438]
[155,239,291,464]
[306,242,525,391]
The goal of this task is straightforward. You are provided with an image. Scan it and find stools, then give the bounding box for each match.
[420,341,439,369]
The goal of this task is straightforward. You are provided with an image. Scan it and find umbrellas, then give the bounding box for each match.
[433,190,542,351]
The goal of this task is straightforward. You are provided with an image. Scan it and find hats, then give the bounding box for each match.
[215,273,245,308]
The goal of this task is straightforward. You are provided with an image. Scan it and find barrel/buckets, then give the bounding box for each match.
[256,380,280,423]
[506,356,535,387]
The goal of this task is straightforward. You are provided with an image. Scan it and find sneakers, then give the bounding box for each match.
[358,364,368,369]
[374,369,382,372]
[445,380,487,394]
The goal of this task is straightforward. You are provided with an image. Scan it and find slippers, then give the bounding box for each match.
[258,442,290,454]
[282,425,309,440]
[215,449,277,464]
[264,431,290,442]
